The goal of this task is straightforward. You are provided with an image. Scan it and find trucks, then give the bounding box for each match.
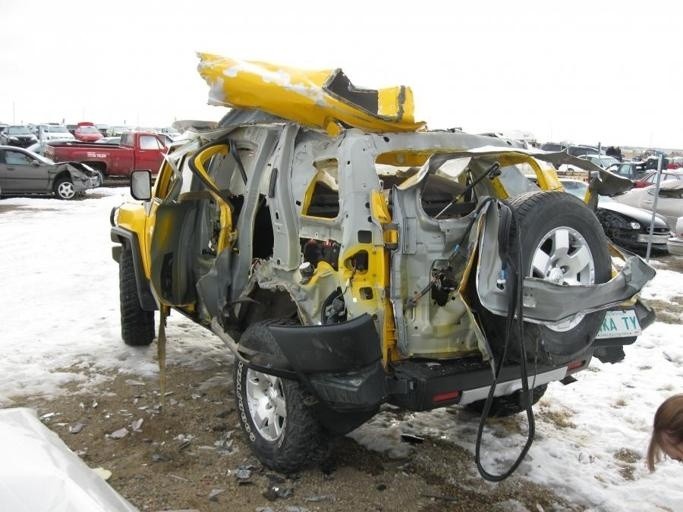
[43,130,168,176]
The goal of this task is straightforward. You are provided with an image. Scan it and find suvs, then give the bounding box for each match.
[109,49,655,475]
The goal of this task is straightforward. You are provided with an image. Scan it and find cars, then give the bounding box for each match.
[525,146,683,247]
[0,145,103,200]
[1,122,181,144]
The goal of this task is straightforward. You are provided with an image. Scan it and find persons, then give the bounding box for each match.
[646,393,682,474]
